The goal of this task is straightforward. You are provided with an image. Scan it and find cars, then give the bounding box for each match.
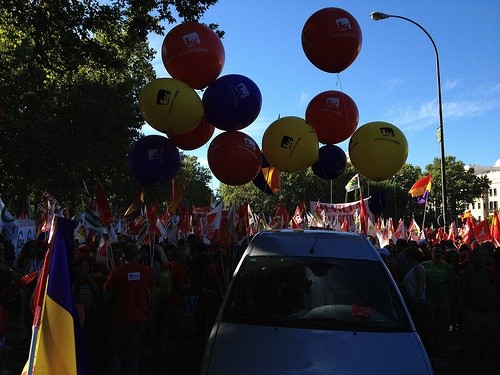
[200,231,434,375]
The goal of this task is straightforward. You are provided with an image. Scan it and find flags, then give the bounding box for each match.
[19,215,81,374]
[0,153,499,266]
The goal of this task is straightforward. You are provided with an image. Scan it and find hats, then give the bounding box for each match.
[419,239,427,245]
[377,248,390,256]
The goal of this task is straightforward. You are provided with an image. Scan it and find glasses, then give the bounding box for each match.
[0,249,5,252]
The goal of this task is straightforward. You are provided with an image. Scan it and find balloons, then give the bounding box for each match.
[126,6,408,215]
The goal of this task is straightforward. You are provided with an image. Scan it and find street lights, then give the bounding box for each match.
[369,12,448,234]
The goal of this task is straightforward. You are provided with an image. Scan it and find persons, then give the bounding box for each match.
[102,243,157,375]
[0,226,500,375]
